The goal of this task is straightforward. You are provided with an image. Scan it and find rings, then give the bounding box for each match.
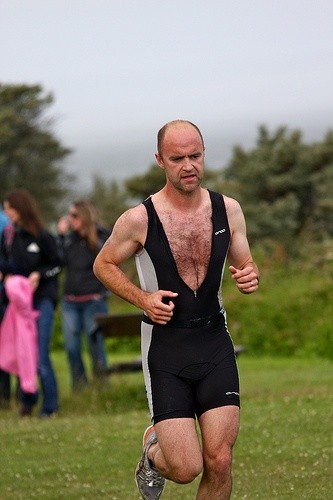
[251,282,255,287]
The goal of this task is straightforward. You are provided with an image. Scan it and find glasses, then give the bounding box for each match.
[71,213,80,218]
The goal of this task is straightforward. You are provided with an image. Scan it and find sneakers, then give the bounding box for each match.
[134,424,166,500]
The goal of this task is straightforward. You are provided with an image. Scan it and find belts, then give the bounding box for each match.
[63,294,100,302]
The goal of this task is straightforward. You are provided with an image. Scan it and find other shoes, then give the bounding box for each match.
[41,410,56,418]
[20,409,30,418]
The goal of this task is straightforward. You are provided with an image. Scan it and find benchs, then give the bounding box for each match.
[88,313,248,388]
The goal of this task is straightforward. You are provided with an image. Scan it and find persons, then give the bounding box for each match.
[1,189,111,418]
[93,121,261,500]
